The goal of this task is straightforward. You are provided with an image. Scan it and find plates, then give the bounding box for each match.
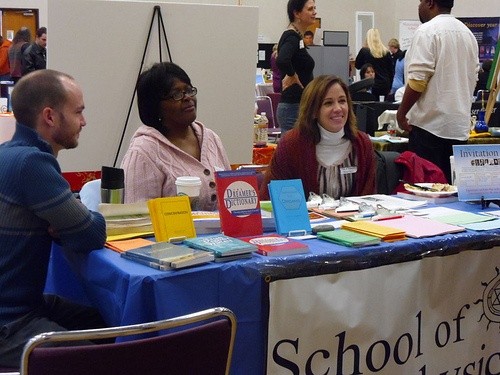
[404,181,458,199]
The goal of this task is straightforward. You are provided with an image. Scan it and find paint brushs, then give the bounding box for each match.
[399,178,431,191]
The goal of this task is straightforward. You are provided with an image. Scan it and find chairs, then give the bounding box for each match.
[267,93,282,128]
[256,96,275,129]
[18,304,237,375]
[374,150,389,196]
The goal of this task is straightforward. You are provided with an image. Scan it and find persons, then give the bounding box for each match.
[0,26,48,98]
[260,74,378,200]
[355,28,408,103]
[121,62,232,212]
[270,0,318,132]
[0,69,116,370]
[396,0,480,184]
[473,60,495,95]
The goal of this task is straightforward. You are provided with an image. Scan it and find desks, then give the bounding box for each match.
[368,134,410,153]
[467,131,500,144]
[43,193,500,375]
[256,82,274,96]
[377,109,405,133]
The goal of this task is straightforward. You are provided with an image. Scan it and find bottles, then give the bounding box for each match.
[253,112,268,143]
[474,111,488,133]
[388,120,397,138]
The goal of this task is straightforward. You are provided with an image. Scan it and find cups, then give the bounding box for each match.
[175,177,201,211]
[471,118,476,130]
[100,166,126,206]
[379,95,385,102]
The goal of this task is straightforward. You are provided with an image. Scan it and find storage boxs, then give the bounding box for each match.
[253,146,275,165]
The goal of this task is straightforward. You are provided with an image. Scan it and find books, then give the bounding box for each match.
[97,171,404,272]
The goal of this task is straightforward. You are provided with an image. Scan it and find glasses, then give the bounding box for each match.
[162,86,198,101]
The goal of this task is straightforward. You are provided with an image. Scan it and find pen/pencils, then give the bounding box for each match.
[337,210,359,213]
[373,215,404,221]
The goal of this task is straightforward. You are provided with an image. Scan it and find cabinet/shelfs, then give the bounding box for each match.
[307,44,350,90]
